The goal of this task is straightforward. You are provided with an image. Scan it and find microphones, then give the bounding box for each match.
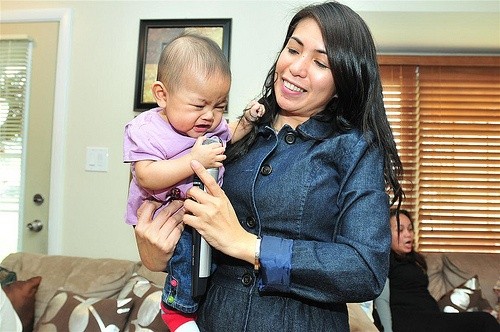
[189,138,221,299]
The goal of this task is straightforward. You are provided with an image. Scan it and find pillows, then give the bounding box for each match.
[437,275,482,313]
[117,272,170,332]
[34,288,135,332]
[2,276,42,332]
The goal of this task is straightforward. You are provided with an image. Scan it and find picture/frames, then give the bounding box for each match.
[133,18,232,113]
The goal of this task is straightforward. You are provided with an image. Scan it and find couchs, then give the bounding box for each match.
[0,252,168,328]
[346,251,500,332]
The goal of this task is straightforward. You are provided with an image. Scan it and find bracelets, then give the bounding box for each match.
[253,236,260,272]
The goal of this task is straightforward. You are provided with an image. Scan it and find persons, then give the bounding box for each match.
[134,2,404,332]
[122,34,266,332]
[372,207,500,332]
